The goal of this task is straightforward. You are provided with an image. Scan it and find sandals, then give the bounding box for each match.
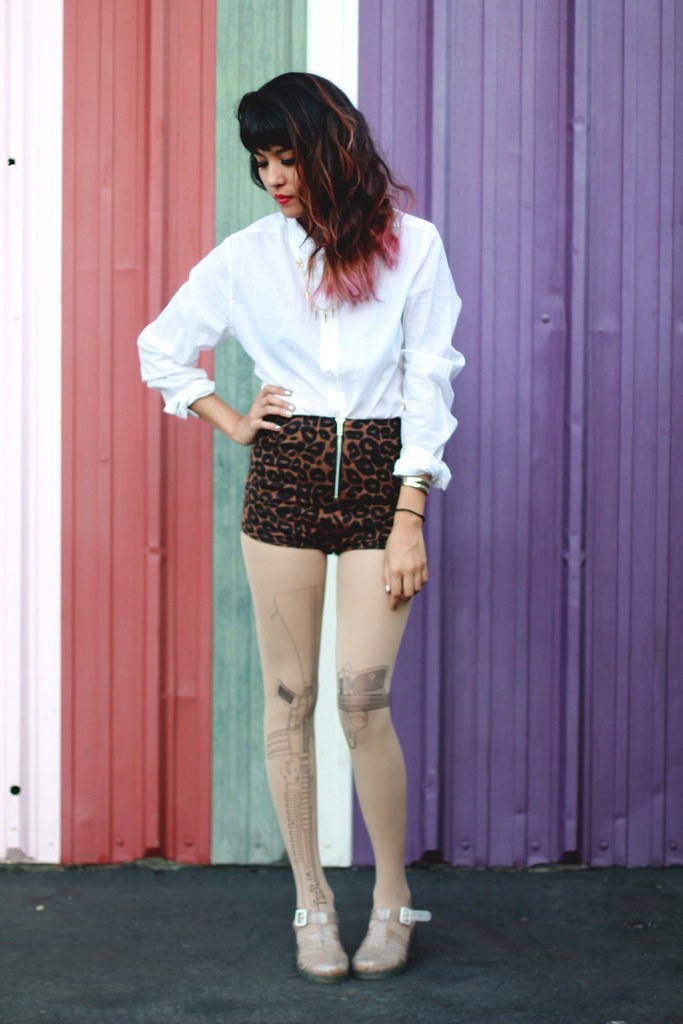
[292,908,349,984]
[351,905,432,981]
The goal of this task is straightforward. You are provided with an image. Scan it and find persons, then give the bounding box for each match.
[137,72,469,985]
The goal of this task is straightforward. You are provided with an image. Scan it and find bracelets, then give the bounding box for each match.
[401,476,433,494]
[394,507,426,521]
